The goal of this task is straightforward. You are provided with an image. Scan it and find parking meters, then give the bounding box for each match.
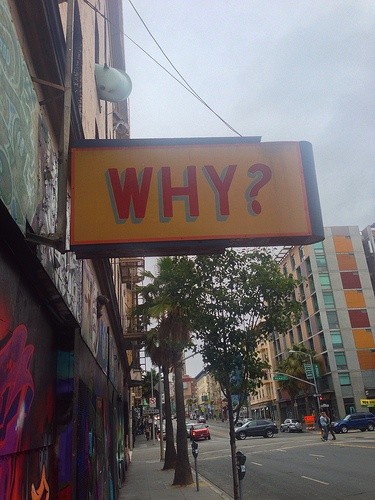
[236,451,246,499]
[191,439,199,491]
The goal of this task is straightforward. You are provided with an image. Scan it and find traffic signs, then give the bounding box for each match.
[312,393,322,397]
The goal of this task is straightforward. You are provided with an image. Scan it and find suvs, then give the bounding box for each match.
[280,418,302,433]
[331,412,375,433]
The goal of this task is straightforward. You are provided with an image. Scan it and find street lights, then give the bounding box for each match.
[289,350,321,416]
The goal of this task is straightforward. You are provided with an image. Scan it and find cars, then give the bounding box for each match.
[189,423,210,440]
[186,423,195,437]
[234,419,278,440]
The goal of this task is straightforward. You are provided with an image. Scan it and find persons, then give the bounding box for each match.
[318,412,336,440]
[140,418,160,440]
